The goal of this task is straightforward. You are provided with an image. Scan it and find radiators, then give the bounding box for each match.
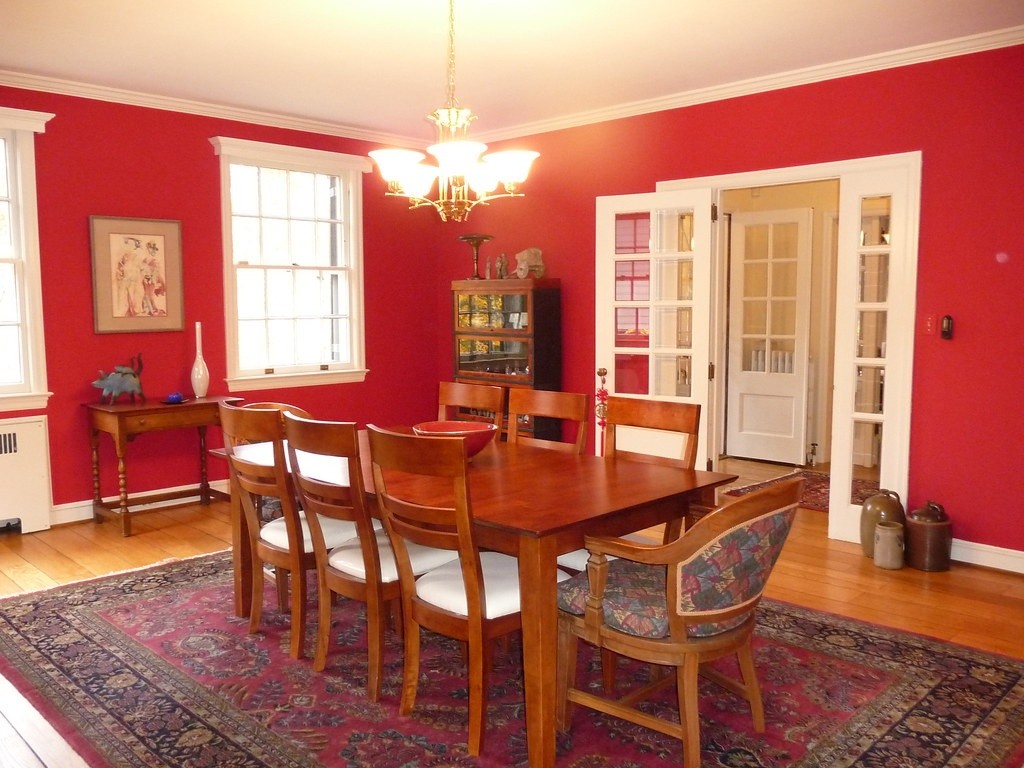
[751,350,792,372]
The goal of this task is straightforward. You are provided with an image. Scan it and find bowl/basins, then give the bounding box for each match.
[413,420,499,465]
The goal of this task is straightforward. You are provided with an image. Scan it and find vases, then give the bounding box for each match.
[191,320,209,398]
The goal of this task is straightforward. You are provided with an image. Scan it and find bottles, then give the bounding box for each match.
[190,321,210,399]
[861,488,905,558]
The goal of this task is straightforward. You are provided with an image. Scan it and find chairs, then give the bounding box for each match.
[218,399,403,662]
[281,409,467,702]
[437,381,504,440]
[233,403,311,611]
[506,388,590,453]
[557,475,804,768]
[557,394,702,576]
[367,424,571,756]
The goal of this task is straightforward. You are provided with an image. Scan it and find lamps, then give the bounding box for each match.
[367,0,542,222]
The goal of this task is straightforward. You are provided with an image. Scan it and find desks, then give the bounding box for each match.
[81,396,243,537]
[208,430,739,768]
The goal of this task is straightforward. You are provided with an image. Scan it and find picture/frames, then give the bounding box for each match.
[88,215,184,333]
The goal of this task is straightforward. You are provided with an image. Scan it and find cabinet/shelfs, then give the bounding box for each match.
[451,278,564,439]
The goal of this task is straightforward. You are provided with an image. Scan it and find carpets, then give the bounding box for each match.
[0,545,1024,768]
[720,468,880,512]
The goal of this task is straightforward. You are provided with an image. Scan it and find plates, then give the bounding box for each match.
[160,398,190,404]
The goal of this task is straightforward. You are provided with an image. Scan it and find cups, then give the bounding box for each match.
[166,392,184,402]
[873,520,905,571]
[905,500,953,572]
